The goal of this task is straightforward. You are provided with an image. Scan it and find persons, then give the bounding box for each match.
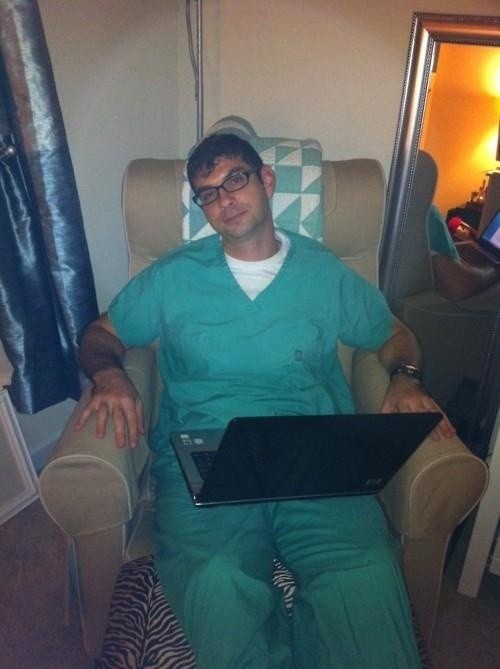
[74,130,457,669]
[416,148,463,264]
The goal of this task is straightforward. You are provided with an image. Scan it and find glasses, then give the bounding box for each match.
[193,171,254,207]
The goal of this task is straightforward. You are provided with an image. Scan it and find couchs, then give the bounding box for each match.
[392,149,499,460]
[35,160,492,662]
[99,556,435,665]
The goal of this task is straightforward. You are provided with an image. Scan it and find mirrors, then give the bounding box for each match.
[377,10,499,603]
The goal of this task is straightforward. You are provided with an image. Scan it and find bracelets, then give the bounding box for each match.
[389,364,421,379]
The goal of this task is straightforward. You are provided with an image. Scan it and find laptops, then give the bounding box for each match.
[169,412,444,507]
[455,206,500,270]
